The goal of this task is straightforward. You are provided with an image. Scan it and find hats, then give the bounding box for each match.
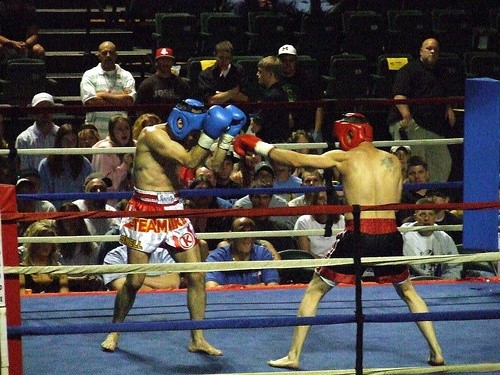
[254,161,274,175]
[155,47,175,60]
[278,44,296,56]
[32,92,54,108]
[16,178,30,187]
[391,145,411,154]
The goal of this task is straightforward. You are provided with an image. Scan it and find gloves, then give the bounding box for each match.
[198,105,233,151]
[217,104,247,151]
[233,134,275,157]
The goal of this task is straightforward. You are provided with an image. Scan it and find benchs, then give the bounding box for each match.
[0,0,500,280]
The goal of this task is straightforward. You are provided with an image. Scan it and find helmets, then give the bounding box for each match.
[331,112,373,152]
[167,99,208,146]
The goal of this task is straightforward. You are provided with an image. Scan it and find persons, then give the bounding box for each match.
[101,99,246,356]
[0,0,465,295]
[233,113,444,369]
